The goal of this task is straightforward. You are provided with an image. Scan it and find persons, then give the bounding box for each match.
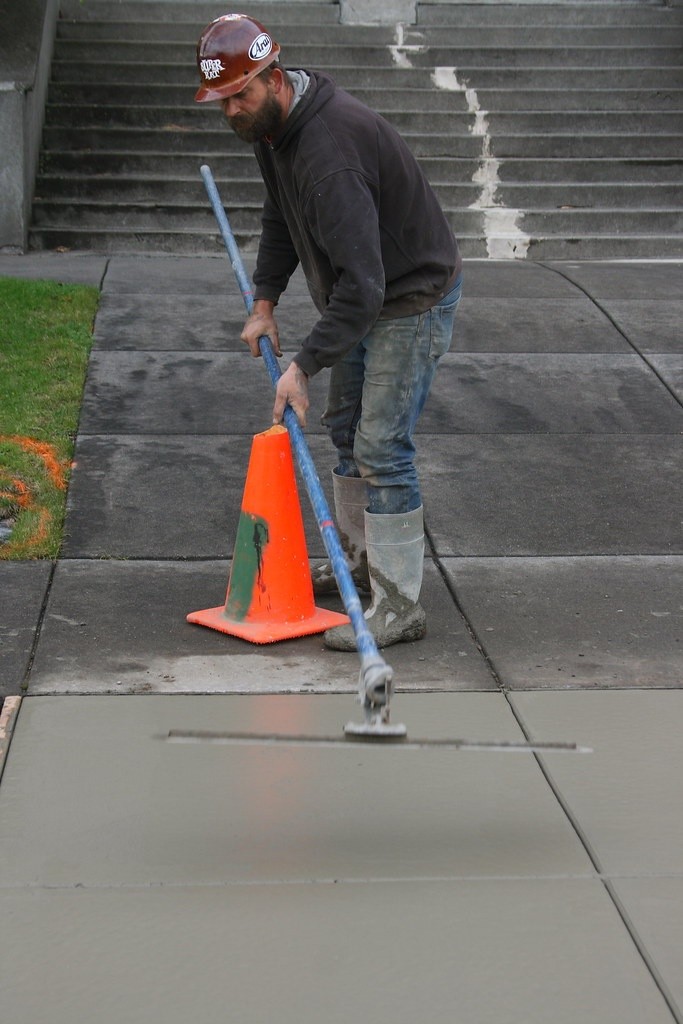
[195,13,462,651]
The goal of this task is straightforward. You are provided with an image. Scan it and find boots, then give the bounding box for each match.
[309,481,370,602]
[318,500,432,650]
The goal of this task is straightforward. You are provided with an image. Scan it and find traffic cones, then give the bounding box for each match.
[186,425,350,644]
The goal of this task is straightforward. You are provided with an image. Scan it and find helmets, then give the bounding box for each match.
[192,13,281,103]
